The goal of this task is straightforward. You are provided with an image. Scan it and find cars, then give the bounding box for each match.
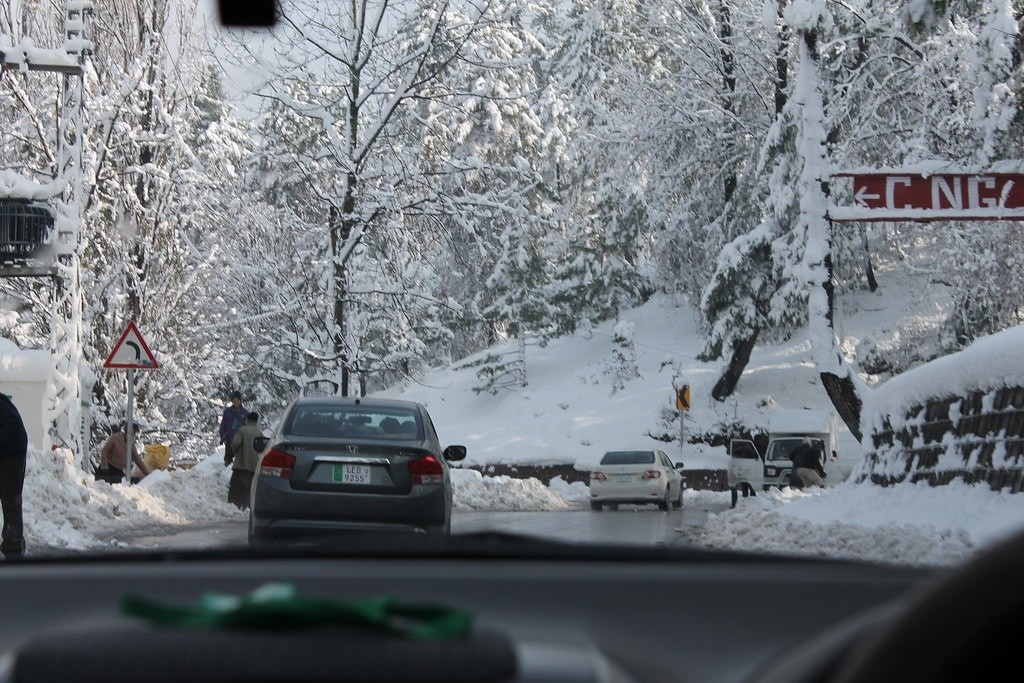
[589,448,684,510]
[248,396,467,552]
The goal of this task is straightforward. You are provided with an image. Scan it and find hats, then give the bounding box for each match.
[247,412,258,421]
[124,422,140,432]
[231,391,241,401]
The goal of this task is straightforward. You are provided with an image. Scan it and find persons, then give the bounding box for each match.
[95,423,149,485]
[733,436,756,497]
[218,391,249,467]
[227,411,267,510]
[789,437,828,490]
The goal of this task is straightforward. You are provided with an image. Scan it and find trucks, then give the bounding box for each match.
[727,412,837,493]
